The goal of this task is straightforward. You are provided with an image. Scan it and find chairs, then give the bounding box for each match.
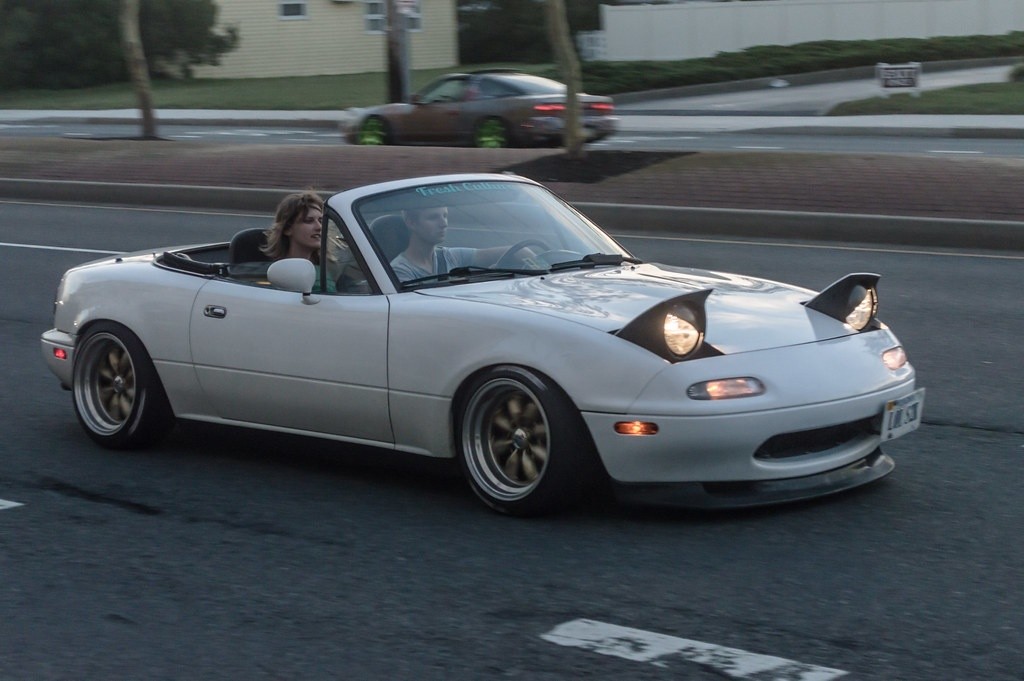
[228,228,272,276]
[369,215,411,260]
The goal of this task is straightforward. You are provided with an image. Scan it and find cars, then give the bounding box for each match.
[337,64,618,151]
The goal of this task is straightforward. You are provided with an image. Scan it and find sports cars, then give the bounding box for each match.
[38,168,926,521]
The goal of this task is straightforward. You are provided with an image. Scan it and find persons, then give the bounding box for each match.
[258,192,341,297]
[389,206,538,286]
